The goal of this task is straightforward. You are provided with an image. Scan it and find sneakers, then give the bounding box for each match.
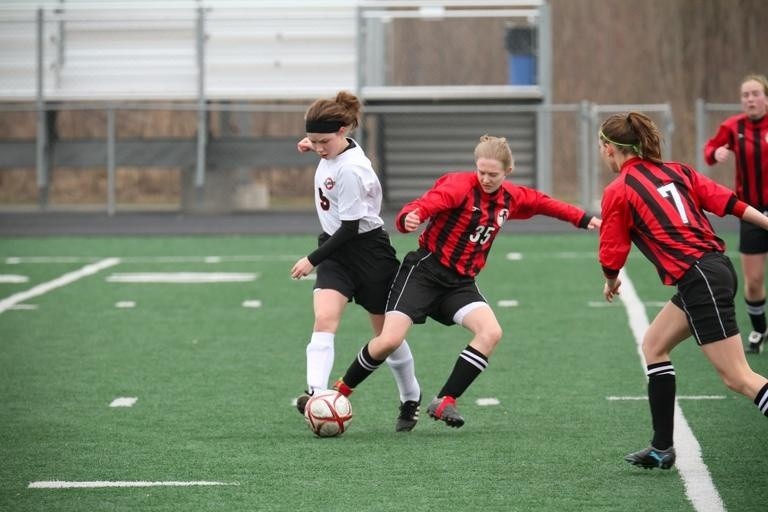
[743,327,768,354]
[621,440,678,470]
[426,394,466,429]
[295,390,312,416]
[393,389,423,433]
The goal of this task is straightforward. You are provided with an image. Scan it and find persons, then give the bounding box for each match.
[333,134,604,428]
[705,72,768,357]
[288,89,424,433]
[597,111,768,471]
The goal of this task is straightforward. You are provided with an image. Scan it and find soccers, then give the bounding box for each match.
[305,390,353,437]
